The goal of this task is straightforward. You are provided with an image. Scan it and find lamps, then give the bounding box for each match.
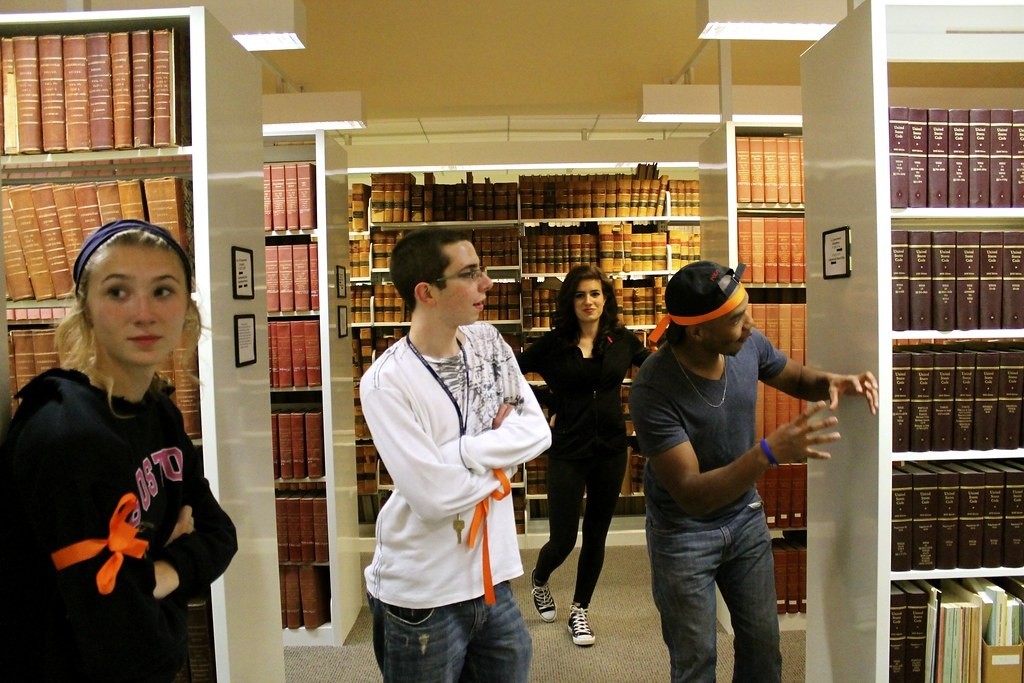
[636,84,803,128]
[261,90,368,137]
[695,0,848,43]
[68,0,309,53]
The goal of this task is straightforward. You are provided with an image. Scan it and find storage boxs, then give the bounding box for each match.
[982,639,1023,683]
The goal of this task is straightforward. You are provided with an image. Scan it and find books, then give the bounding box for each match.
[735,137,808,615]
[261,159,326,630]
[1,27,217,683]
[346,170,704,528]
[889,106,1024,683]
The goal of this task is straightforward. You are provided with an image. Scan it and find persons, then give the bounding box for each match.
[627,259,878,683]
[357,225,554,683]
[515,262,669,645]
[0,219,238,683]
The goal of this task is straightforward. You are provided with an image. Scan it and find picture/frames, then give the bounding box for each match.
[231,245,255,300]
[233,314,257,369]
[337,305,348,338]
[822,225,853,281]
[336,265,347,299]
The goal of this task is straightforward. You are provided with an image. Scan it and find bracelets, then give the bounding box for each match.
[759,438,780,466]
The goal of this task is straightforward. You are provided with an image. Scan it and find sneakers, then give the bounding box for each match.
[531,569,557,623]
[567,601,595,645]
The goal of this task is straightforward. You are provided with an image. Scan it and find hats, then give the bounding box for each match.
[662,260,746,315]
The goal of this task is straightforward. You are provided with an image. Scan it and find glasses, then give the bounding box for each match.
[431,266,487,282]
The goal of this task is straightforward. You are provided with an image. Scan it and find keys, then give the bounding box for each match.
[453,514,465,543]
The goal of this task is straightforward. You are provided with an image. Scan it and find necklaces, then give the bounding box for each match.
[673,344,729,408]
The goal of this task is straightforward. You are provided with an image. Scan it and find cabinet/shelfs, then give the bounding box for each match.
[0,8,288,683]
[368,196,531,554]
[348,230,372,328]
[263,131,363,646]
[694,121,807,636]
[516,190,702,550]
[797,5,1024,683]
